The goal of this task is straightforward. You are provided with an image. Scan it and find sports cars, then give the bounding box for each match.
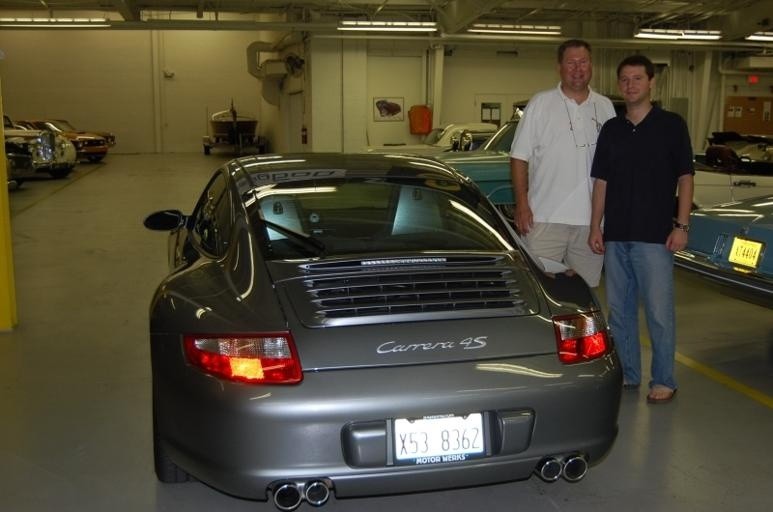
[674,129,773,219]
[139,152,622,512]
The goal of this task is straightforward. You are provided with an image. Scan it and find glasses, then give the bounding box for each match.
[571,118,600,148]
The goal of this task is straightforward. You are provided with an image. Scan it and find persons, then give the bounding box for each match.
[506,39,616,291]
[586,54,696,405]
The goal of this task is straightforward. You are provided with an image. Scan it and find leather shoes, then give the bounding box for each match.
[646,384,677,404]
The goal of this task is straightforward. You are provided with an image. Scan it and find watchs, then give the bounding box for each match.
[672,220,689,232]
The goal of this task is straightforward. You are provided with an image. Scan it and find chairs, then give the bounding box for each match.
[259,195,312,242]
[373,182,451,251]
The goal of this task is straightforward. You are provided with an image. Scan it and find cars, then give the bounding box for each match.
[5,108,118,192]
[368,117,518,231]
[674,194,773,300]
